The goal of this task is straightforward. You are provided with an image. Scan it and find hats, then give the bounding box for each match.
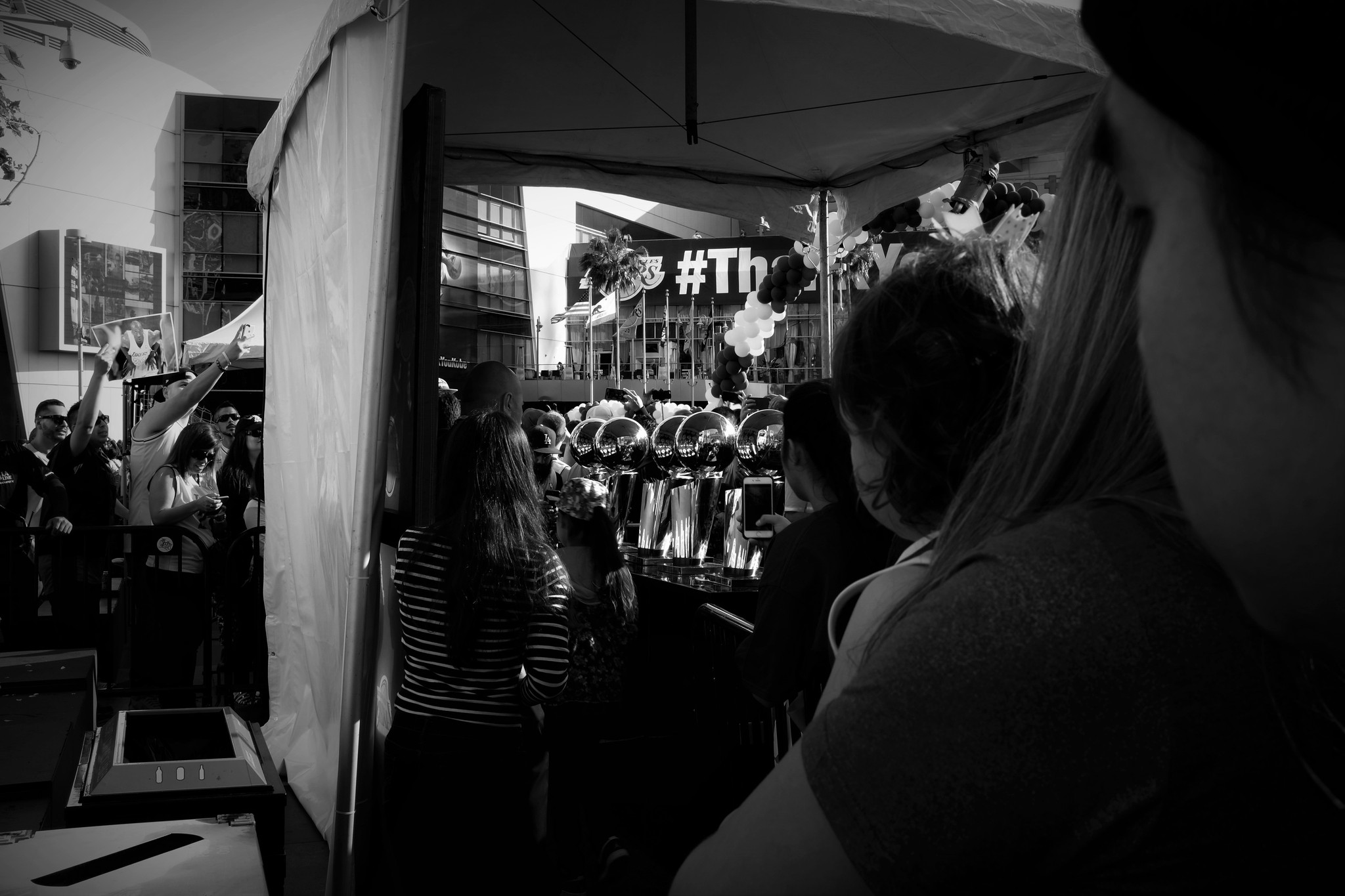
[152,367,199,402]
[526,424,562,454]
[556,477,612,521]
[522,408,546,427]
[235,414,263,435]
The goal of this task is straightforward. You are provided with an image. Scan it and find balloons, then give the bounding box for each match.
[565,179,1058,424]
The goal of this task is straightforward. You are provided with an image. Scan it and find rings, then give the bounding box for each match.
[210,504,214,509]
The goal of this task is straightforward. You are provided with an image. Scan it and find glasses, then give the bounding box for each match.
[39,415,69,427]
[189,449,215,461]
[215,413,241,424]
[95,415,109,426]
[245,429,262,438]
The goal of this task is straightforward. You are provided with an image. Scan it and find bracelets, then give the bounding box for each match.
[216,358,228,372]
[222,352,231,365]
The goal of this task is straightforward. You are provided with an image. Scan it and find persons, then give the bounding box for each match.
[350,255,1030,896]
[70,250,154,345]
[1077,0,1345,666]
[120,321,162,378]
[0,323,266,713]
[673,64,1345,896]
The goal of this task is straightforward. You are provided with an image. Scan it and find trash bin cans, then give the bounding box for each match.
[66,705,290,896]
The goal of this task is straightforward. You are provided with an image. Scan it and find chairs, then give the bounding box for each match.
[532,367,709,379]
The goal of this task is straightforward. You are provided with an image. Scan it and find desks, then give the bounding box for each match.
[682,369,702,379]
[548,371,563,380]
[594,370,611,380]
[642,369,654,379]
[574,371,587,380]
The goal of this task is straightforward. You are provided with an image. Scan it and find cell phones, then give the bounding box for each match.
[743,476,774,539]
[213,496,229,506]
[242,324,250,338]
[543,489,560,510]
[721,390,742,404]
[604,387,629,402]
[652,390,671,400]
[747,396,771,409]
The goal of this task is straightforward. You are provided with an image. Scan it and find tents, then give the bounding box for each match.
[181,293,264,370]
